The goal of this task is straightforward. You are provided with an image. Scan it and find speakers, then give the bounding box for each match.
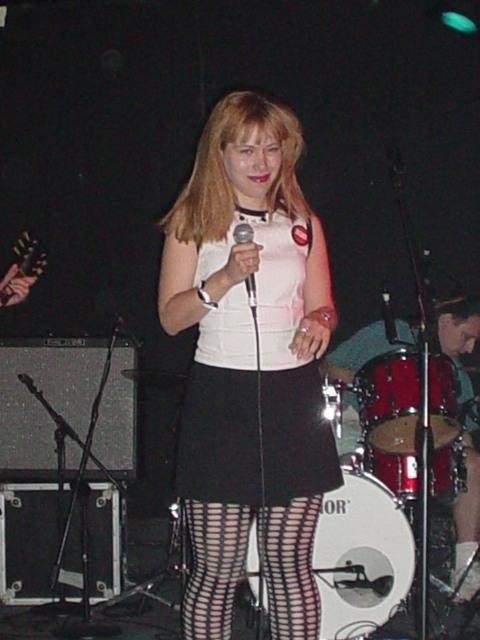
[0,335,139,482]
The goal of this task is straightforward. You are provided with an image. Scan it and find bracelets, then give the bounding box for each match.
[196,280,219,311]
[304,304,339,330]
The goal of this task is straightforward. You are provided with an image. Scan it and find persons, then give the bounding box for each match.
[319,283,480,607]
[156,90,341,640]
[0,262,38,307]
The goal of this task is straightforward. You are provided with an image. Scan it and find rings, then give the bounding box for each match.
[299,326,309,333]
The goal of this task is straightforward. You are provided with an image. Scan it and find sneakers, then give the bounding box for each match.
[449,561,479,604]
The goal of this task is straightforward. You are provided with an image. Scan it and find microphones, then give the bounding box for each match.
[113,311,147,353]
[381,291,397,340]
[234,224,257,317]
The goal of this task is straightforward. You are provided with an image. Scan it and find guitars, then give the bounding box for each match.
[0,230,46,314]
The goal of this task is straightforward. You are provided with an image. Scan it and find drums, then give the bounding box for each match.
[243,455,419,640]
[362,442,468,509]
[353,343,465,459]
